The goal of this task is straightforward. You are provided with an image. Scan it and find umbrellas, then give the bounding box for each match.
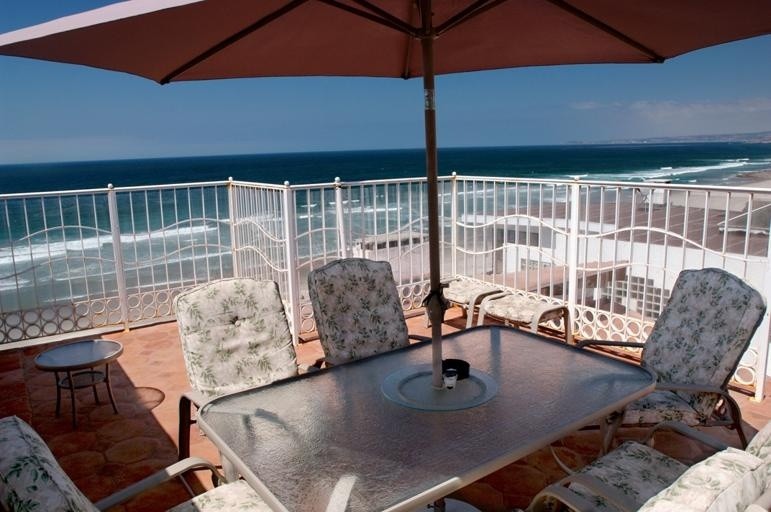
[0,1,771,391]
